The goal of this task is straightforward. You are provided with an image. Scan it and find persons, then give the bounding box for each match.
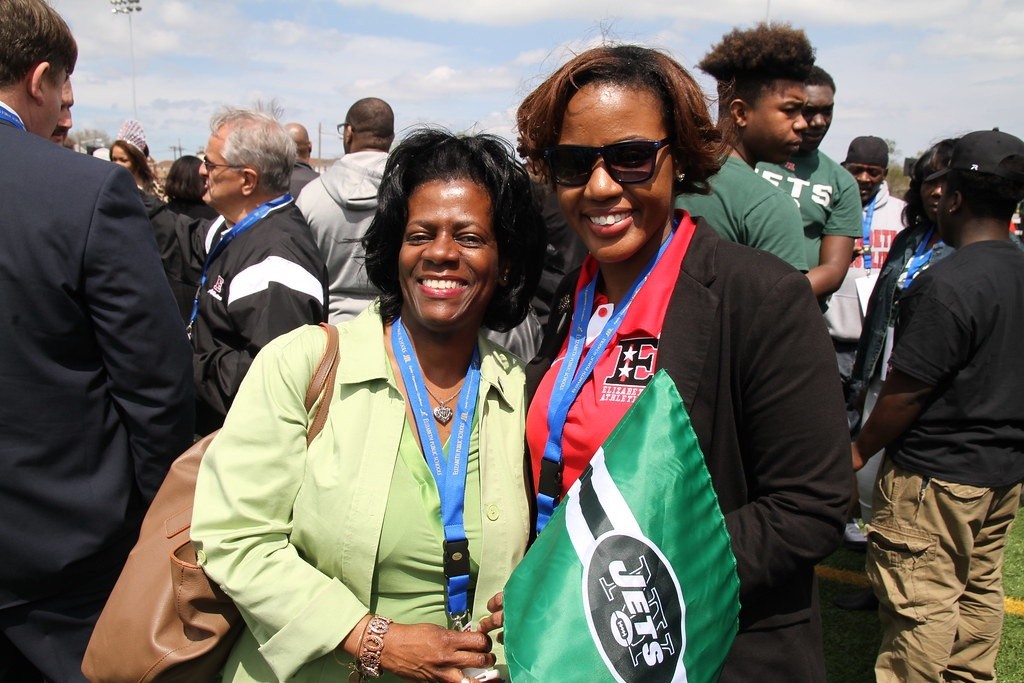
[185,97,329,448]
[47,113,207,285]
[671,25,808,276]
[841,138,956,431]
[193,127,547,683]
[293,97,395,329]
[822,136,909,546]
[850,127,1024,683]
[514,46,865,683]
[751,67,864,298]
[0,0,193,683]
[278,122,323,206]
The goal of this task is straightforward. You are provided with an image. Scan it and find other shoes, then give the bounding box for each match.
[846,589,880,610]
[845,522,868,548]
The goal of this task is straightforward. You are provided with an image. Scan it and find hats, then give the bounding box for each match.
[840,136,889,168]
[925,127,1024,182]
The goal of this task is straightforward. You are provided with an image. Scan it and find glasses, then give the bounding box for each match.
[542,135,672,187]
[337,123,355,134]
[204,156,259,185]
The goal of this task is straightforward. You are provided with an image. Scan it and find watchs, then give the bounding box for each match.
[359,613,392,679]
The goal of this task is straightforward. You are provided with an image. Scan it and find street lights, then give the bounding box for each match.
[111,0,142,123]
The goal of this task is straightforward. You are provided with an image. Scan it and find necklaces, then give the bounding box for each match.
[424,381,465,426]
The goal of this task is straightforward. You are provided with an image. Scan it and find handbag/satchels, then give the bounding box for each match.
[77,322,341,683]
